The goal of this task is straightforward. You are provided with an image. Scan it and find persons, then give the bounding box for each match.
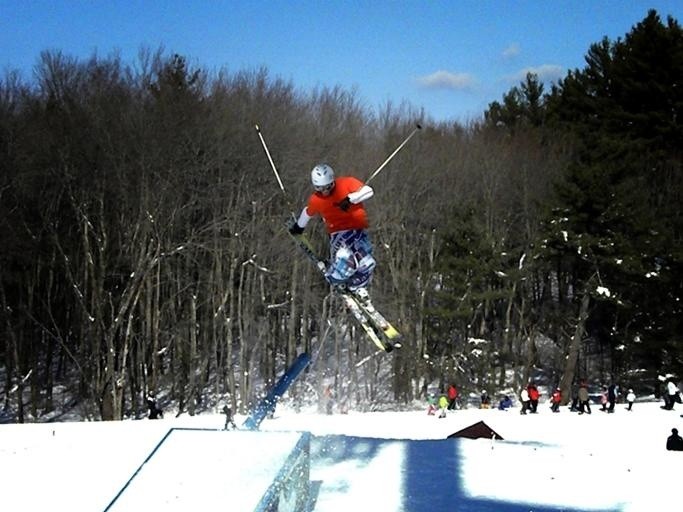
[478,372,683,415]
[145,389,158,419]
[290,164,377,293]
[220,405,236,431]
[428,382,457,419]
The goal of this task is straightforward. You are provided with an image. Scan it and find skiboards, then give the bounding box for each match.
[285,220,402,351]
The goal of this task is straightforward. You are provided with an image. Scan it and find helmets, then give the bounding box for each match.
[311,165,335,195]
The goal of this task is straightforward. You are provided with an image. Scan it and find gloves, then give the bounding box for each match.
[290,222,304,234]
[339,197,351,211]
